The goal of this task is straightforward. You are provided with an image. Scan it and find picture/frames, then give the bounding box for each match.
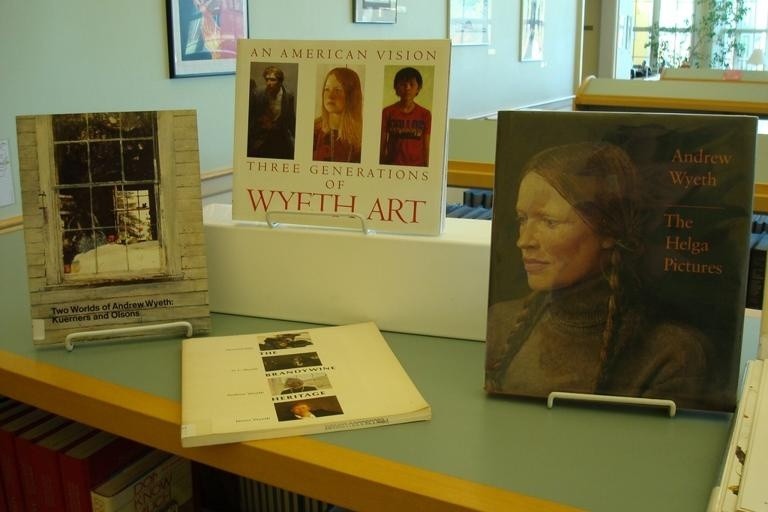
[351,0,398,26]
[165,0,250,80]
[446,1,492,47]
[519,1,545,62]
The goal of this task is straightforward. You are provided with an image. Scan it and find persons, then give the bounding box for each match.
[250,66,294,155]
[380,66,431,167]
[313,66,361,162]
[485,140,719,408]
[269,337,344,422]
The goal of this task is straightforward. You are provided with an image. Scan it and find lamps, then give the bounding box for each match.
[746,48,766,71]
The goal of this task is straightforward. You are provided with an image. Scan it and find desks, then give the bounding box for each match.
[447,116,768,214]
[2,228,765,511]
[574,66,768,115]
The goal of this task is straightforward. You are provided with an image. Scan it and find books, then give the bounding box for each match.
[484,107,758,414]
[746,213,768,310]
[14,107,215,350]
[445,187,493,221]
[705,357,768,511]
[180,319,433,452]
[0,396,195,512]
